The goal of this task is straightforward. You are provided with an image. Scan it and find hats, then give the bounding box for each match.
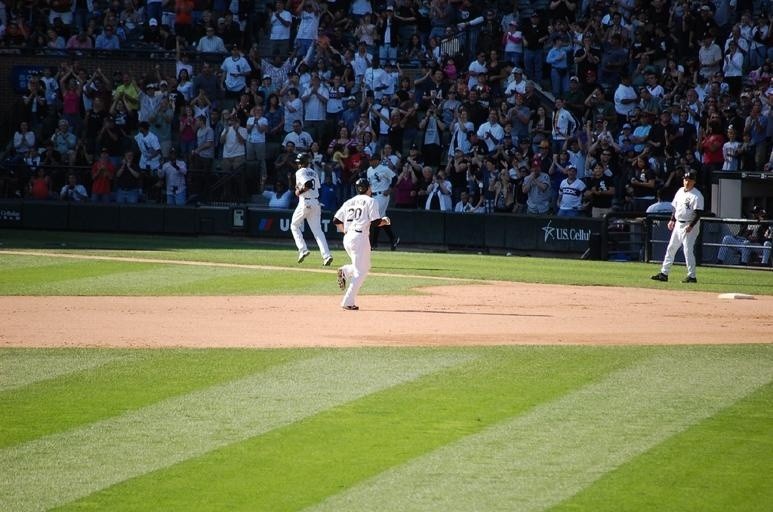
[0,0,773,170]
[355,178,370,195]
[681,172,698,182]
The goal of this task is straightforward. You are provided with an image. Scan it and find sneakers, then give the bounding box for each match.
[342,302,359,310]
[321,254,333,267]
[371,243,379,250]
[680,276,697,283]
[650,272,669,282]
[297,249,310,264]
[336,267,346,291]
[390,236,400,250]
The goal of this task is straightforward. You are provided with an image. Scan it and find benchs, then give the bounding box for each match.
[732,252,771,265]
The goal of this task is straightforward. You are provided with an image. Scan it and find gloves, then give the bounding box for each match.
[382,216,390,225]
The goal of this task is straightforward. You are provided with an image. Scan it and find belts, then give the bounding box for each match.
[678,221,690,223]
[304,197,319,200]
[354,230,362,233]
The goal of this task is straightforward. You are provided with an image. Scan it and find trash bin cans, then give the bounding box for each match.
[229,205,249,238]
[646,202,674,261]
